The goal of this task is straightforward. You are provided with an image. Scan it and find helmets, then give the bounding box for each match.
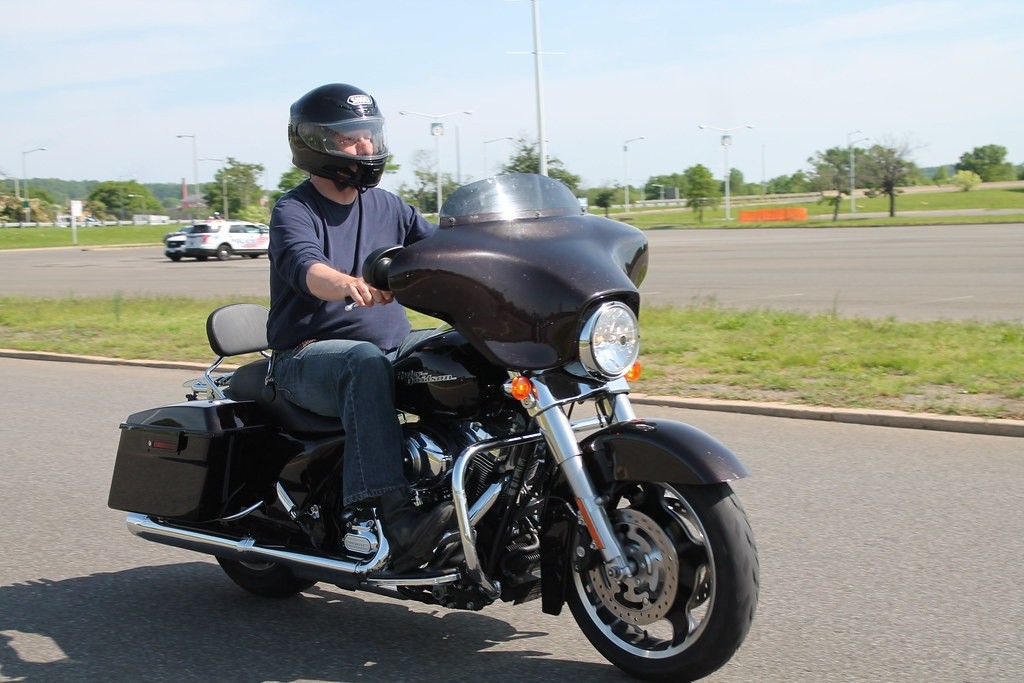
[288,84,388,187]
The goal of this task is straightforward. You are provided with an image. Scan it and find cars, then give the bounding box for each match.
[161,224,190,261]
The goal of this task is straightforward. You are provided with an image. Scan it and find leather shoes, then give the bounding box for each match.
[381,501,454,576]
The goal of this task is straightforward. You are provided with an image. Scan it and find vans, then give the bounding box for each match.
[185,216,269,261]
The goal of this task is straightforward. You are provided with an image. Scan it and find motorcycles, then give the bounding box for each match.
[108,170,761,683]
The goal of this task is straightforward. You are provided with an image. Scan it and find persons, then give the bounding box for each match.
[267,83,455,566]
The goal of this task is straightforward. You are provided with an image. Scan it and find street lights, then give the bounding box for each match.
[398,110,474,218]
[481,137,515,178]
[849,136,870,221]
[22,147,46,207]
[621,135,646,213]
[196,156,228,223]
[177,134,200,216]
[696,124,756,221]
